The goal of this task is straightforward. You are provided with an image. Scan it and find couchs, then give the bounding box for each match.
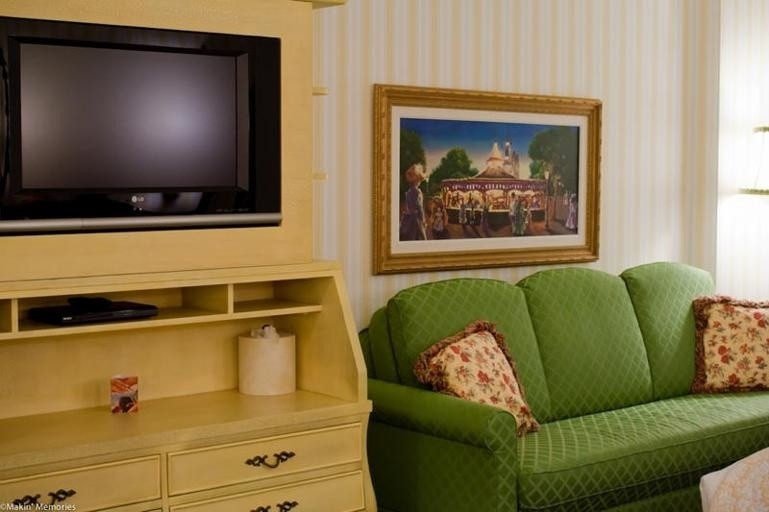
[353,261,769,512]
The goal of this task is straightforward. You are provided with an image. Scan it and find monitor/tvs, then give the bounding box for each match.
[0,15,282,246]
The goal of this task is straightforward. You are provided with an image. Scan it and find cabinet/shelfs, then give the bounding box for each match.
[2,258,379,512]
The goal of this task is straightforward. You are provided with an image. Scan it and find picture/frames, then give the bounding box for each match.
[373,84,612,274]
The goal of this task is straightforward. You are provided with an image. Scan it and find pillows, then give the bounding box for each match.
[688,294,769,393]
[412,318,540,439]
